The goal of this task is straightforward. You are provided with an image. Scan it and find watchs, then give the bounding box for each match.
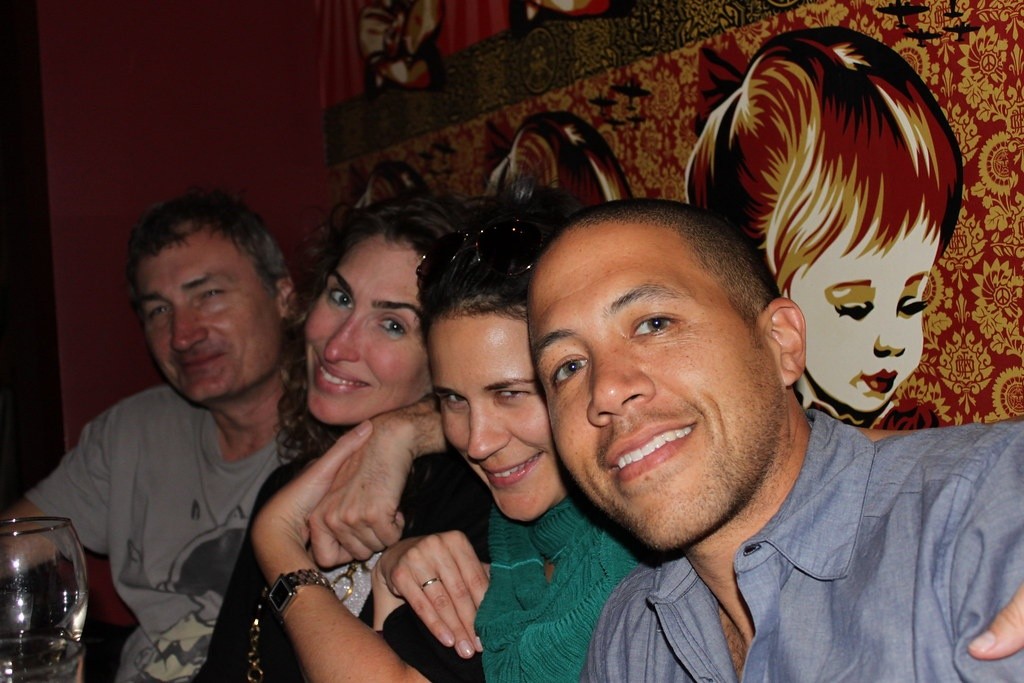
[267,570,331,616]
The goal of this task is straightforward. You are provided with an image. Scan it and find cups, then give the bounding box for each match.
[0,516,88,683]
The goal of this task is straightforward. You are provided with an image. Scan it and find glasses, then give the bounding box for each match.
[417,213,567,293]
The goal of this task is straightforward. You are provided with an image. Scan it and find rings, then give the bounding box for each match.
[421,577,440,591]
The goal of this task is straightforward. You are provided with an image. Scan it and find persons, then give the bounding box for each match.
[0,187,446,683]
[525,199,1024,683]
[371,199,1024,683]
[193,199,493,683]
